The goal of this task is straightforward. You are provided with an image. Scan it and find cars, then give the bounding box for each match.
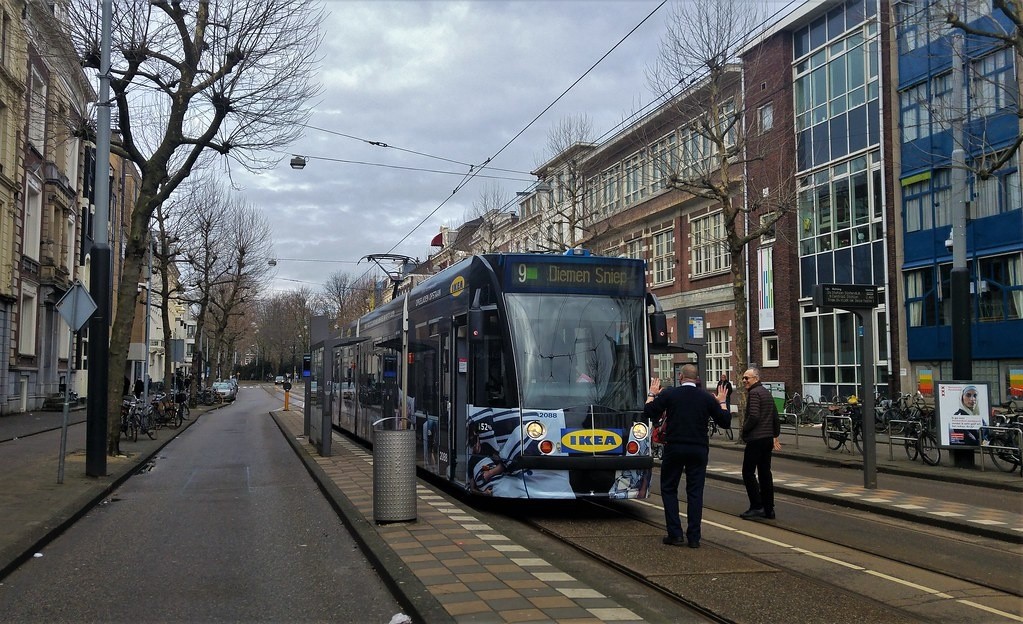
[333,382,356,399]
[275,376,285,385]
[211,379,239,402]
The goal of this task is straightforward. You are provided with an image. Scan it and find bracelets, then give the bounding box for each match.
[720,402,727,404]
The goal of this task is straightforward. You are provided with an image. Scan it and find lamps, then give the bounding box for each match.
[535,182,552,193]
[267,259,276,266]
[290,157,306,169]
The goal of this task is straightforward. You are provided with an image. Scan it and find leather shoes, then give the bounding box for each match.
[740,508,765,519]
[688,541,700,548]
[663,535,684,544]
[764,510,775,519]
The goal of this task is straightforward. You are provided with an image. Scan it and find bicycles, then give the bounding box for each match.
[784,399,824,427]
[831,391,942,466]
[989,401,1023,477]
[121,384,222,443]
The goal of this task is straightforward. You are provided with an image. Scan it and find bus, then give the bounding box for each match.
[314,248,668,499]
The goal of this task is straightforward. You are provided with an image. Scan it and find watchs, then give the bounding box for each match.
[648,393,655,398]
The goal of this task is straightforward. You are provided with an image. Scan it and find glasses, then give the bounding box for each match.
[742,376,755,381]
[965,392,977,398]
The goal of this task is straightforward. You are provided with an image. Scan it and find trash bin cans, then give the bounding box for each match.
[371,416,418,524]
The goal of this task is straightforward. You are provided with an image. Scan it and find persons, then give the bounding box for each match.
[716,374,733,413]
[135,376,143,399]
[123,375,130,396]
[954,386,979,417]
[739,366,782,521]
[148,374,152,397]
[467,420,506,494]
[642,364,729,550]
[177,376,191,393]
